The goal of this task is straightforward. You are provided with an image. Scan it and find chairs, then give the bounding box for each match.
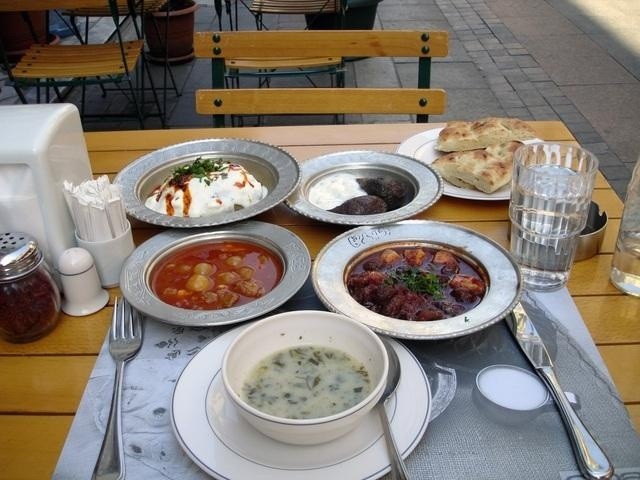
[3,2,186,133]
[197,2,350,128]
[183,30,447,126]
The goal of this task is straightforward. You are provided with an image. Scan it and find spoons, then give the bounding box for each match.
[377,333,411,479]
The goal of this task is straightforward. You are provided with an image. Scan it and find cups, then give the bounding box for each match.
[74,219,136,288]
[508,142,599,293]
[473,365,581,428]
[609,155,640,297]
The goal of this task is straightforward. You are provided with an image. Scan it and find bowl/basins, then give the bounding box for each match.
[221,310,390,446]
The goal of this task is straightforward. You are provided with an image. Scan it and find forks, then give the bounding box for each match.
[90,295,143,480]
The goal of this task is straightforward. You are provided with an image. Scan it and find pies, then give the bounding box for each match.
[428,115,537,195]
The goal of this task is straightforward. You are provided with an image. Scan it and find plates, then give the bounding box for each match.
[397,127,547,201]
[414,302,560,374]
[170,318,432,480]
[112,136,301,228]
[283,149,444,225]
[119,220,312,328]
[418,359,458,423]
[311,218,524,342]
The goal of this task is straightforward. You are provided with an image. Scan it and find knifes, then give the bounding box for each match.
[505,302,615,479]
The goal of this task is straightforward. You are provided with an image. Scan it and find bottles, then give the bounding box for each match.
[60,247,110,317]
[0,231,62,344]
